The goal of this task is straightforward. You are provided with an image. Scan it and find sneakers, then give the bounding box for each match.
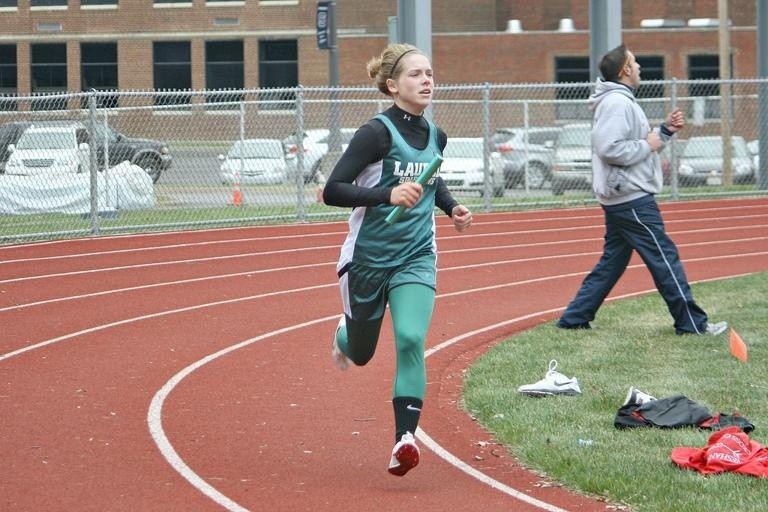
[623,386,657,407]
[518,360,583,396]
[706,321,729,337]
[332,314,351,369]
[388,430,420,476]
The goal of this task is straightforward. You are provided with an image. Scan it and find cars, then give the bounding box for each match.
[4,119,99,176]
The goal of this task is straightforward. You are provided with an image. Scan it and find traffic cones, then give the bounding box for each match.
[224,169,251,206]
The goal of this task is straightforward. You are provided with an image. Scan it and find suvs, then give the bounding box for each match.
[0,117,176,189]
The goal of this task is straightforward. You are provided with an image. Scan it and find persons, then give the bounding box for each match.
[318,41,472,476]
[557,42,728,338]
